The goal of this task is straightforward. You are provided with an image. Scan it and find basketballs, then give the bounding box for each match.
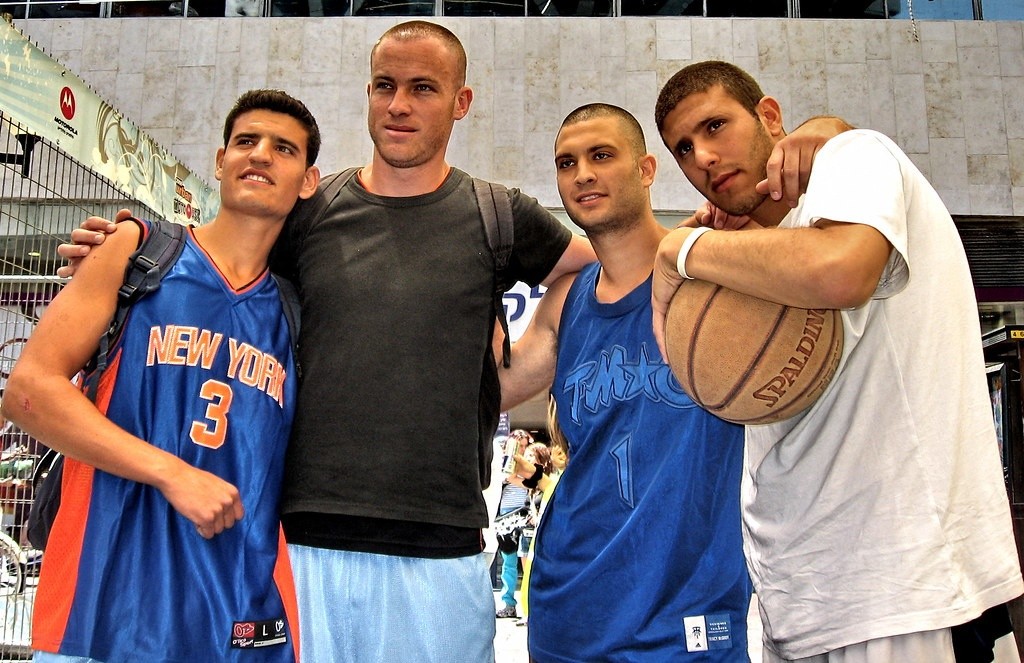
[660,277,847,428]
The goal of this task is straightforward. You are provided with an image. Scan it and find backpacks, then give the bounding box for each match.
[26,222,302,552]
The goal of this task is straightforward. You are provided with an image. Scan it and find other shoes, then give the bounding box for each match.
[496,603,518,618]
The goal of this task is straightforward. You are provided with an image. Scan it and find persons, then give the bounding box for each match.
[57,22,750,663]
[2,89,320,663]
[0,397,15,587]
[492,103,854,663]
[481,430,568,626]
[651,61,1024,663]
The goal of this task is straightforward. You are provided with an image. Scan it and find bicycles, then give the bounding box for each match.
[0,535,27,593]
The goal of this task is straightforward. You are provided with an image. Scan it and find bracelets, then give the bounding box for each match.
[522,463,543,489]
[677,227,714,279]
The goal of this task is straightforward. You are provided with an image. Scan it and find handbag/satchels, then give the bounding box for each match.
[493,506,532,553]
